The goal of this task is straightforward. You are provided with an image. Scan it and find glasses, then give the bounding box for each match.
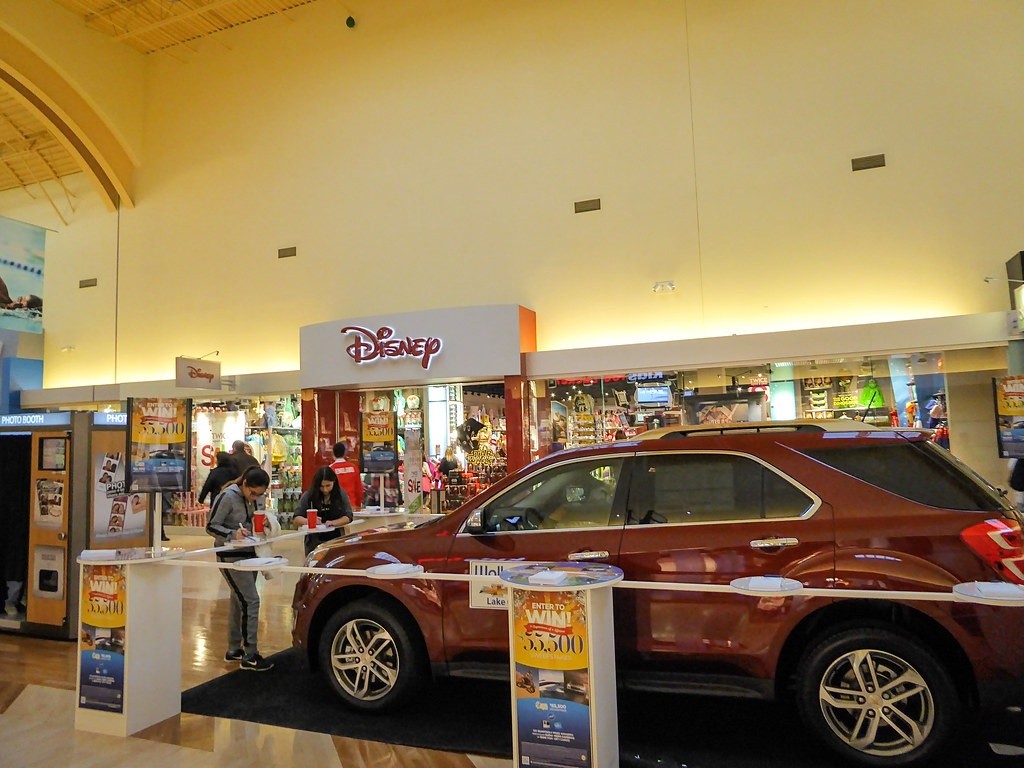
[247,487,266,498]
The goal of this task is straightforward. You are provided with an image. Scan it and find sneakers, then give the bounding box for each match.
[224,649,246,662]
[240,654,275,672]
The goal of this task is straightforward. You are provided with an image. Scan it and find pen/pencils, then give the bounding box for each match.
[237,522,244,530]
[315,515,323,526]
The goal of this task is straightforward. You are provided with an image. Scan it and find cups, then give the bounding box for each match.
[307,501,318,529]
[253,510,265,533]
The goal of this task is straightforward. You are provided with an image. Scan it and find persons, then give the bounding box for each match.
[329,443,363,512]
[435,447,459,477]
[205,466,275,671]
[292,466,353,559]
[4,581,26,615]
[197,452,239,509]
[228,440,260,477]
[523,670,533,693]
[422,453,432,498]
[37,450,147,534]
[162,517,170,541]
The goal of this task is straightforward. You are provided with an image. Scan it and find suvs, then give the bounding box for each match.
[289,418,1024,767]
